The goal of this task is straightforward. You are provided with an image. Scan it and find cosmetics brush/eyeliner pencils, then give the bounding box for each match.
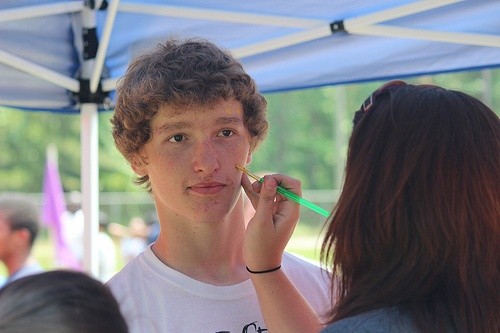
[234,163,330,218]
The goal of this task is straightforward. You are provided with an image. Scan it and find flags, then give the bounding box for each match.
[41,145,82,270]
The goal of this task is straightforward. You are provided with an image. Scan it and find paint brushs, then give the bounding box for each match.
[234,164,332,224]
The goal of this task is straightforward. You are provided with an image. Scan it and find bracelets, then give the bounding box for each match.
[247,266,281,274]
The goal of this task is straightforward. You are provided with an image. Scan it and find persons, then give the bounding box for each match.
[0,270,129,333]
[101,37,350,333]
[62,190,160,286]
[0,193,46,290]
[241,80,500,333]
